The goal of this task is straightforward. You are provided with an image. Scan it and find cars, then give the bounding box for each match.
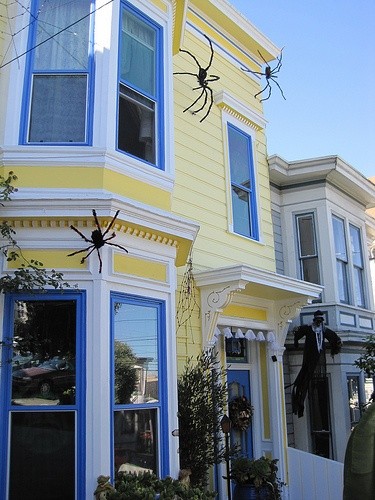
[12,360,76,397]
